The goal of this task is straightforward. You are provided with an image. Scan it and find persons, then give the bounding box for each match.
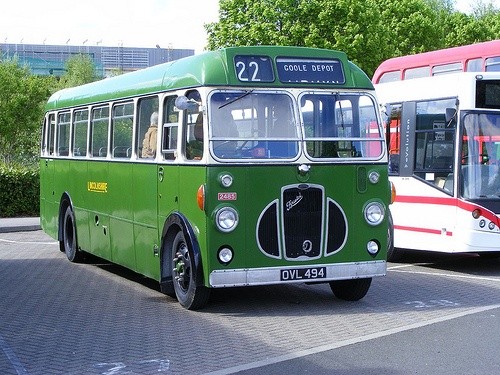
[195,93,240,154]
[268,98,298,157]
[141,112,160,160]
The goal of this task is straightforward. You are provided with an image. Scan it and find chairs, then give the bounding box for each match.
[42,145,140,158]
[195,104,241,152]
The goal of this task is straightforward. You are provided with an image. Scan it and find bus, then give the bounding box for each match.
[372,40,500,84]
[40,46,392,310]
[186,72,500,261]
